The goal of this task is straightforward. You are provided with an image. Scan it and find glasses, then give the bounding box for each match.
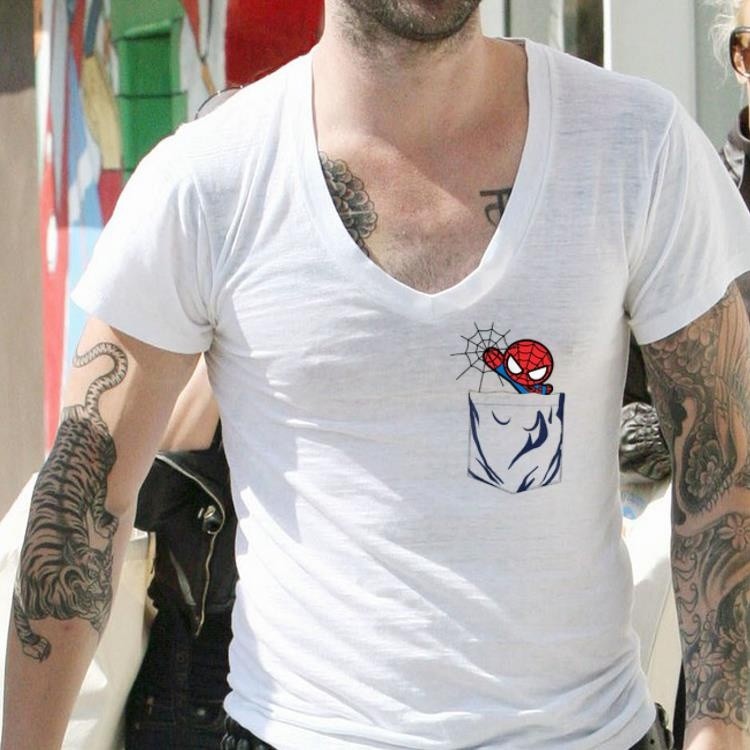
[729,26,750,79]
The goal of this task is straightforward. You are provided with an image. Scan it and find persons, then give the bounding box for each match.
[121,353,236,750]
[0,0,750,750]
[716,0,750,209]
